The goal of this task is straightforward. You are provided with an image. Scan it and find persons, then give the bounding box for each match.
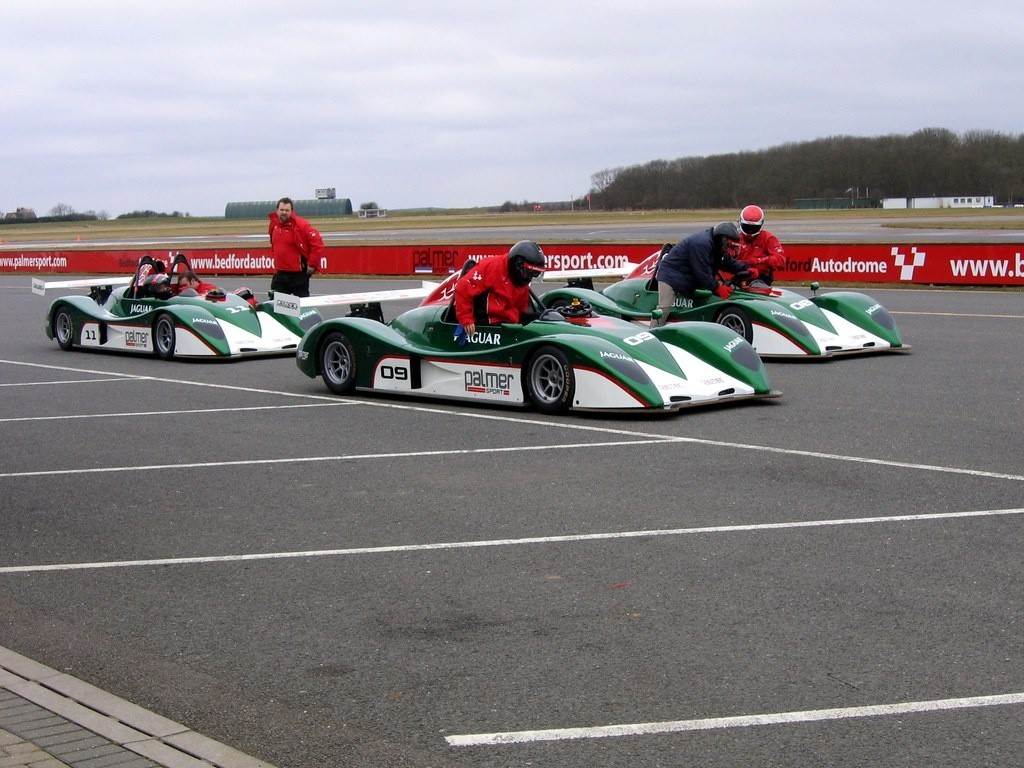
[734,205,785,290]
[145,273,176,299]
[267,196,327,298]
[651,222,753,332]
[455,240,548,335]
[175,273,216,296]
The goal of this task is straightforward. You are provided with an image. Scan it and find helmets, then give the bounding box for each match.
[232,287,258,311]
[142,273,173,300]
[508,240,545,286]
[712,222,740,253]
[739,205,764,241]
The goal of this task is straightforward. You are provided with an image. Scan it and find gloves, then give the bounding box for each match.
[739,258,760,265]
[747,267,759,279]
[715,285,734,300]
[754,265,770,276]
